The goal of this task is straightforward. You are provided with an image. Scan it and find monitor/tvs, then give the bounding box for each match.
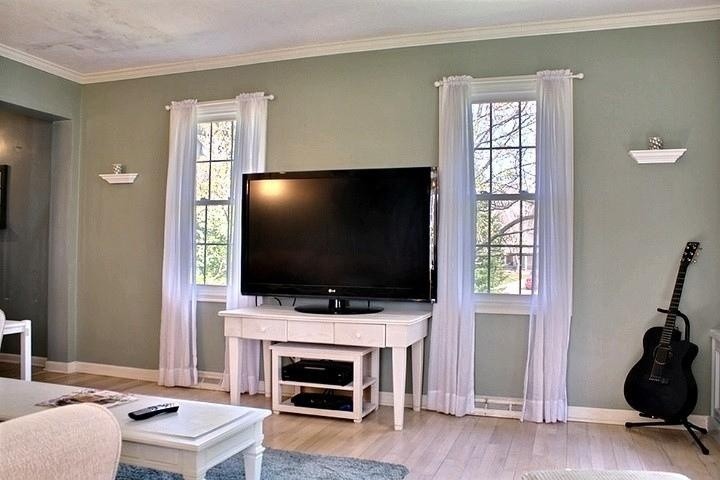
[34,388,140,409]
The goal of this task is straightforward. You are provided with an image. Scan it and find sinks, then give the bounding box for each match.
[0,403,122,480]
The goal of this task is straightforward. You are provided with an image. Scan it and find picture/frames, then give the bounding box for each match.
[3,308,32,381]
[218,303,431,431]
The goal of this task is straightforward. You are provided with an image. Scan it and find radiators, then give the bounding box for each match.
[128,403,179,421]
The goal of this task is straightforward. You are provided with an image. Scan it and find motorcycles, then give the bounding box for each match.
[625,308,709,457]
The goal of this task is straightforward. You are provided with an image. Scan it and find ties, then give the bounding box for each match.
[241,165,437,315]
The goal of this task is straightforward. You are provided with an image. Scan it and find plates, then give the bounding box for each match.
[266,342,380,424]
[708,328,720,446]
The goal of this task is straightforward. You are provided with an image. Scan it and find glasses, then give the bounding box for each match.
[98,163,138,184]
[627,137,688,164]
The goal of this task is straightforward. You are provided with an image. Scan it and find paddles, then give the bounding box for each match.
[520,470,691,480]
[116,443,408,480]
